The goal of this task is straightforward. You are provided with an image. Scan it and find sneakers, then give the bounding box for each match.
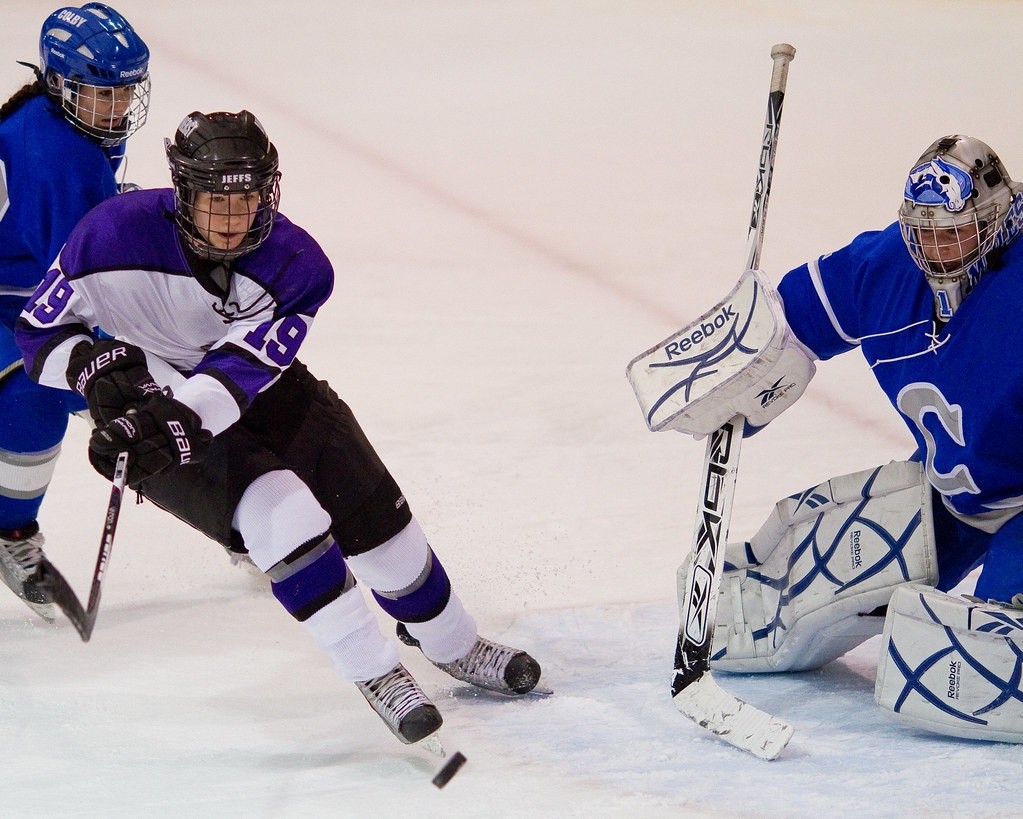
[357,666,448,759]
[396,611,555,698]
[0,521,57,623]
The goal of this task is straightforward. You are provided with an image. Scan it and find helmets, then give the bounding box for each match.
[897,134,1014,322]
[39,2,152,147]
[165,112,281,261]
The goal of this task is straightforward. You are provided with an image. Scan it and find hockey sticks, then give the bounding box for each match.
[35,445,131,644]
[669,43,799,766]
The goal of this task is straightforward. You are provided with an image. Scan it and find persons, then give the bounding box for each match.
[0,3,152,626]
[625,132,1023,744]
[14,112,555,756]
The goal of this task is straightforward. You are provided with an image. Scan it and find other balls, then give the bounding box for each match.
[431,750,468,790]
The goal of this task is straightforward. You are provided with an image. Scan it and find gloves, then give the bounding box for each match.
[65,338,164,428]
[88,387,214,484]
